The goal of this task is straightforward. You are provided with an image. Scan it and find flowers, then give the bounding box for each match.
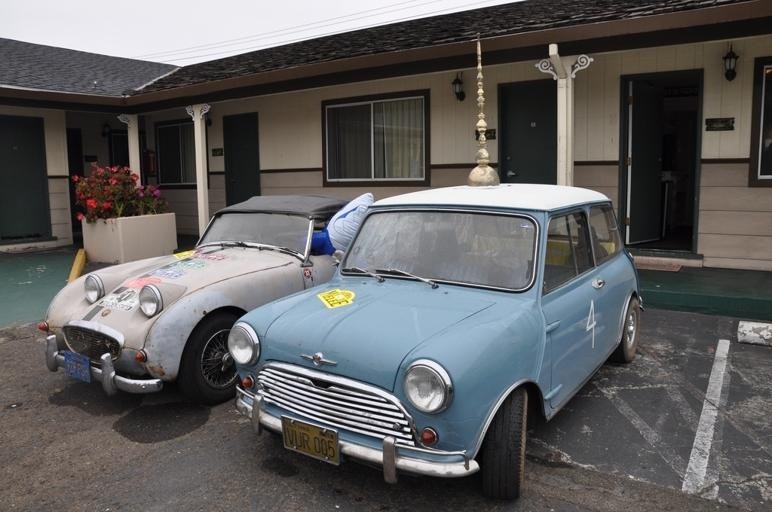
[71,160,169,221]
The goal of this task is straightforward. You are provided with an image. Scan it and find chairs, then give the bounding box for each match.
[568,225,608,270]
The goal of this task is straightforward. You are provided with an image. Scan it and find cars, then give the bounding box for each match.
[37,194,352,405]
[228,183,645,500]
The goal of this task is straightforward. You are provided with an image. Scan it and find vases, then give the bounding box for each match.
[80,212,177,264]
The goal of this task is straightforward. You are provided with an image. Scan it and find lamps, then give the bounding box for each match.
[451,72,466,101]
[722,42,740,81]
